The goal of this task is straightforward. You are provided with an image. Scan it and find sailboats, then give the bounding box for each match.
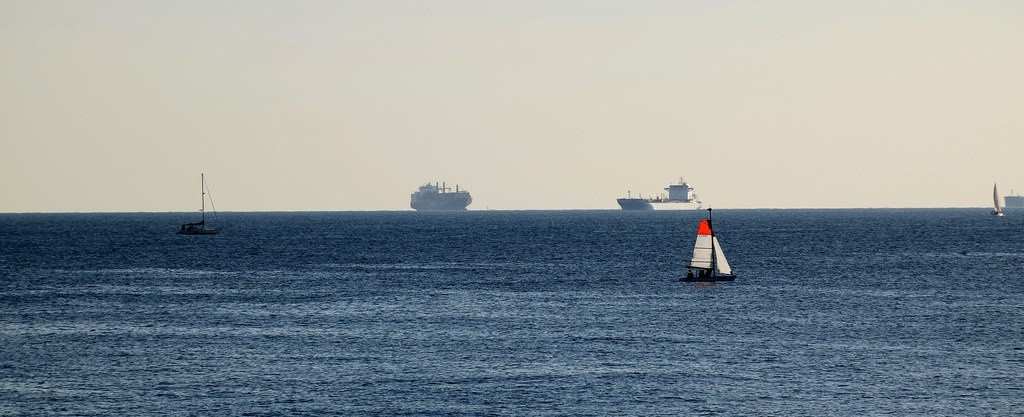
[991,182,1005,216]
[176,171,224,235]
[681,208,738,282]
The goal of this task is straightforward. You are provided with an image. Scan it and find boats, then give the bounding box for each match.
[409,181,473,211]
[616,177,702,211]
